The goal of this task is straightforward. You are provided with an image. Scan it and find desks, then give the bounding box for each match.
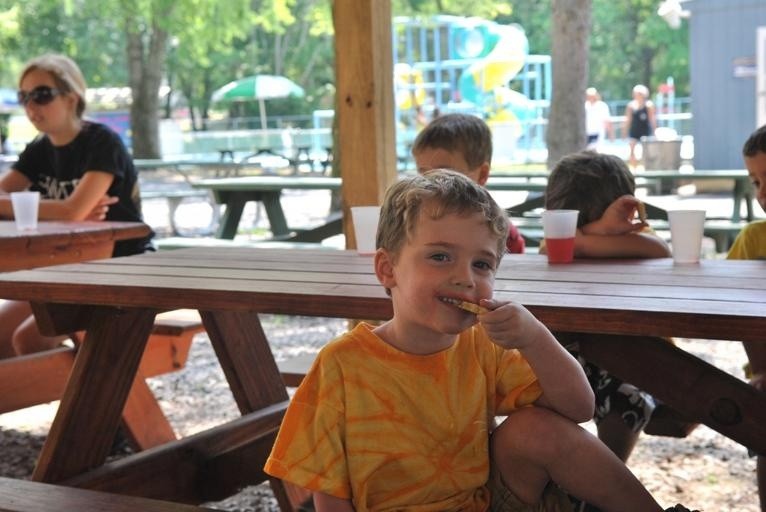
[190,175,342,242]
[485,182,547,216]
[133,158,188,170]
[0,245,766,505]
[631,169,756,223]
[1,218,178,452]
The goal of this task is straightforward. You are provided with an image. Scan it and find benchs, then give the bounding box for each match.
[488,169,550,181]
[631,218,746,254]
[139,188,207,236]
[156,237,337,250]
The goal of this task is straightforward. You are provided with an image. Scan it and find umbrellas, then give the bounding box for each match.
[211,73,305,147]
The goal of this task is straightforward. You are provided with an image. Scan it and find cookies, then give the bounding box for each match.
[459,301,489,315]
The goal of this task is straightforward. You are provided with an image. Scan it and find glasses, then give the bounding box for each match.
[15,85,65,106]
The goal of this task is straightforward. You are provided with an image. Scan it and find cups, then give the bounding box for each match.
[667,209,705,265]
[542,209,580,265]
[11,191,40,232]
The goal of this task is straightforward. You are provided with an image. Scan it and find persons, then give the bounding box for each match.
[262,169,701,512]
[413,114,526,253]
[0,52,158,359]
[726,123,766,392]
[583,86,615,155]
[280,121,301,158]
[620,84,657,168]
[539,148,671,462]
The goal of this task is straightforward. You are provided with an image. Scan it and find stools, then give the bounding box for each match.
[144,318,206,380]
[277,351,319,388]
[509,216,544,241]
[0,476,230,512]
